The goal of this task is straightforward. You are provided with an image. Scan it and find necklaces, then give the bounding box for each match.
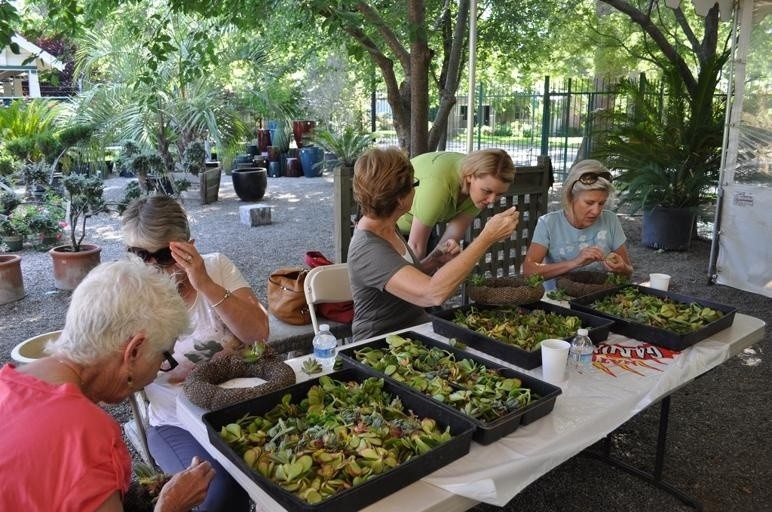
[48,353,88,391]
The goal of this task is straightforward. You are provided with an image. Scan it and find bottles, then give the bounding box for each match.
[313,323,338,374]
[570,328,594,391]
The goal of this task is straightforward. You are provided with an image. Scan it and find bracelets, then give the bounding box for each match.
[208,288,231,308]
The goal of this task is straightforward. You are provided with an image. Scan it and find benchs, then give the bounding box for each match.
[255,305,356,357]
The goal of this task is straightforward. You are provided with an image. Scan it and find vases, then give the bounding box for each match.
[7,326,62,371]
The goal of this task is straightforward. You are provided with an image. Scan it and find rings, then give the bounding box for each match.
[186,254,194,262]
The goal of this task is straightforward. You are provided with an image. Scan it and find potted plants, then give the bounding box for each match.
[47,168,108,293]
[0,213,32,306]
[94,73,305,207]
[588,73,772,256]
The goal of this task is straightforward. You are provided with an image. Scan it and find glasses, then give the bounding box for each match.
[572,172,613,188]
[128,247,176,265]
[159,351,178,372]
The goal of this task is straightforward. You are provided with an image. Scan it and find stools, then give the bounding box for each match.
[119,414,157,477]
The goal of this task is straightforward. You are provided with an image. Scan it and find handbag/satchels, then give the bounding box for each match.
[267,252,353,325]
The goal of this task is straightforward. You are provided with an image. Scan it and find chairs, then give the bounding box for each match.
[300,261,355,348]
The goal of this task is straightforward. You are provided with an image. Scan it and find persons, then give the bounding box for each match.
[120,194,268,511]
[0,257,217,512]
[524,159,634,290]
[390,149,515,263]
[348,146,523,343]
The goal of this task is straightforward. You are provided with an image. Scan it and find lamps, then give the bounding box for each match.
[1,95,92,251]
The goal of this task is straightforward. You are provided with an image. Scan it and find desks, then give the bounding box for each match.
[136,283,772,512]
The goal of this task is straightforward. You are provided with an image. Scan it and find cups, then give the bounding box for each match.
[540,339,570,381]
[649,273,671,292]
